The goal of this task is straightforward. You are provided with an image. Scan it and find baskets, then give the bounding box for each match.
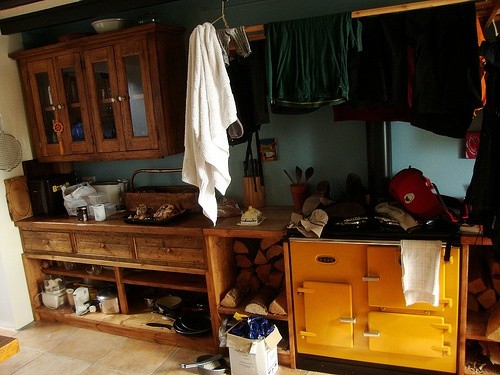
[121,169,201,213]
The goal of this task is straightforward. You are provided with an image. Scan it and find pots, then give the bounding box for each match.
[146,304,212,337]
[180,355,228,375]
[143,296,182,309]
[83,284,128,314]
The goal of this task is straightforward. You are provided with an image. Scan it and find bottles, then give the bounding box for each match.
[52,119,58,144]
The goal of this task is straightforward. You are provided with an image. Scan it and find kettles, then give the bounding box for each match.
[73,286,89,314]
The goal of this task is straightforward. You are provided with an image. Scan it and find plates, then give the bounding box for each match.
[120,208,192,226]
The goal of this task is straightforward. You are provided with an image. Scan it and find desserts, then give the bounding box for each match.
[241,206,262,225]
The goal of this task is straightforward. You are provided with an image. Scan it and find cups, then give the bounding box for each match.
[99,87,111,100]
[290,182,308,210]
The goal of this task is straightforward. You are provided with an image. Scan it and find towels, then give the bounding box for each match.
[181,22,240,229]
[399,238,442,308]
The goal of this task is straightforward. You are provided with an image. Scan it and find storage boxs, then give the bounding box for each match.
[226,319,284,375]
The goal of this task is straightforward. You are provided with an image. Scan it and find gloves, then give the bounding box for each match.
[375,202,418,232]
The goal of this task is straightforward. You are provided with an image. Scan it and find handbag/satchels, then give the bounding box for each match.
[387,168,444,217]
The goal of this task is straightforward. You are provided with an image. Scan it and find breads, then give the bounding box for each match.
[134,202,175,220]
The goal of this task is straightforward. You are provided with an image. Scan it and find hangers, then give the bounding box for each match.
[212,1,236,33]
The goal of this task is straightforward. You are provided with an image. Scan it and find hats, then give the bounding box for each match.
[289,209,329,238]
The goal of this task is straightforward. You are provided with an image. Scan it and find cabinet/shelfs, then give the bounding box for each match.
[289,235,462,374]
[457,232,500,374]
[8,23,188,163]
[203,205,306,370]
[16,212,230,351]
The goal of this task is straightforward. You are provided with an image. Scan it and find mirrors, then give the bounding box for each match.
[367,106,485,208]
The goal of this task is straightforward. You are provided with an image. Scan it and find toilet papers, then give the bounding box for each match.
[93,203,106,222]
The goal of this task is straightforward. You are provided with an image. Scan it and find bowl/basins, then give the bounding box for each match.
[91,18,127,34]
[136,11,161,25]
